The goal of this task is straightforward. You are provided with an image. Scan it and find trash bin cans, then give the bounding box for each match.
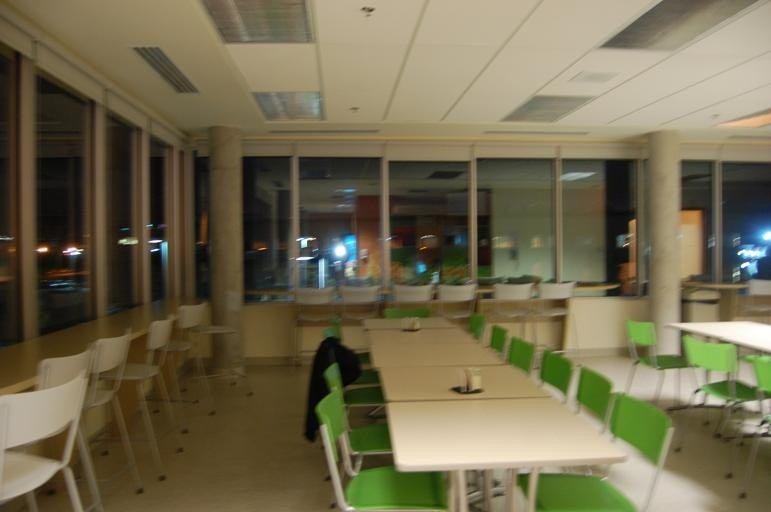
[682,288,722,344]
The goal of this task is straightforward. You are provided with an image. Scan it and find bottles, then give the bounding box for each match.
[400,317,421,330]
[459,367,481,392]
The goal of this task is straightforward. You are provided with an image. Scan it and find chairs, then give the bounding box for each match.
[737,354,771,499]
[625,319,701,408]
[674,334,771,479]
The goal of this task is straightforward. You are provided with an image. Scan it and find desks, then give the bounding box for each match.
[681,282,749,322]
[664,321,771,442]
